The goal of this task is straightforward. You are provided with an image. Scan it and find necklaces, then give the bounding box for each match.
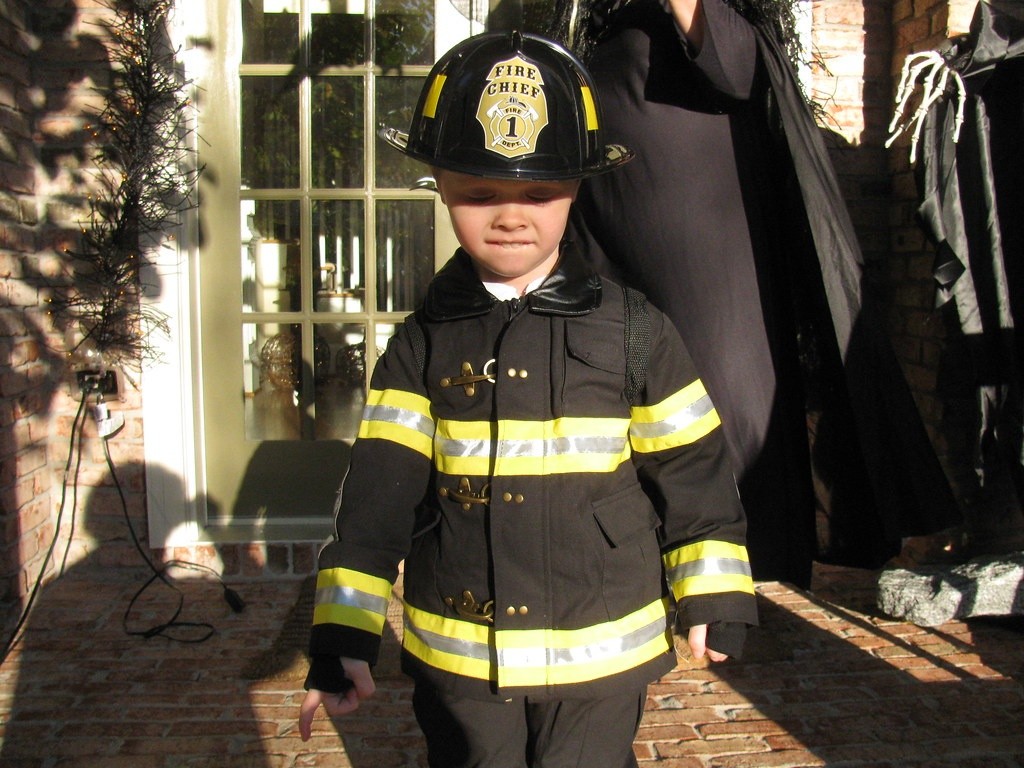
[580,0,630,67]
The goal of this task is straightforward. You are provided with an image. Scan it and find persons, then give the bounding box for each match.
[298,31,760,768]
[487,1,956,587]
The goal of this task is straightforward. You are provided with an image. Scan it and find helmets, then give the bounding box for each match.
[381,28,637,183]
